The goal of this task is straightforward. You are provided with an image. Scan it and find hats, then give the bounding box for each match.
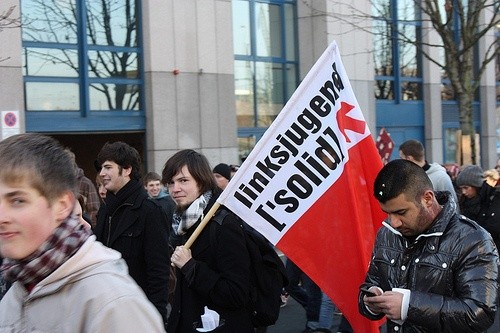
[456,165,483,187]
[213,163,232,181]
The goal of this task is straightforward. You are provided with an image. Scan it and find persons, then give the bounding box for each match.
[358,159,500,333]
[62,139,500,333]
[92,141,166,333]
[0,131,167,333]
[161,147,256,333]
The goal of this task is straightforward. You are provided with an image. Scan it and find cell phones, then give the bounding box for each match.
[361,288,377,297]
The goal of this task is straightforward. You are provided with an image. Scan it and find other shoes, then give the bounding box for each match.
[280,288,290,308]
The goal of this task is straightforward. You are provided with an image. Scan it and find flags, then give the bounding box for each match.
[216,40,387,333]
